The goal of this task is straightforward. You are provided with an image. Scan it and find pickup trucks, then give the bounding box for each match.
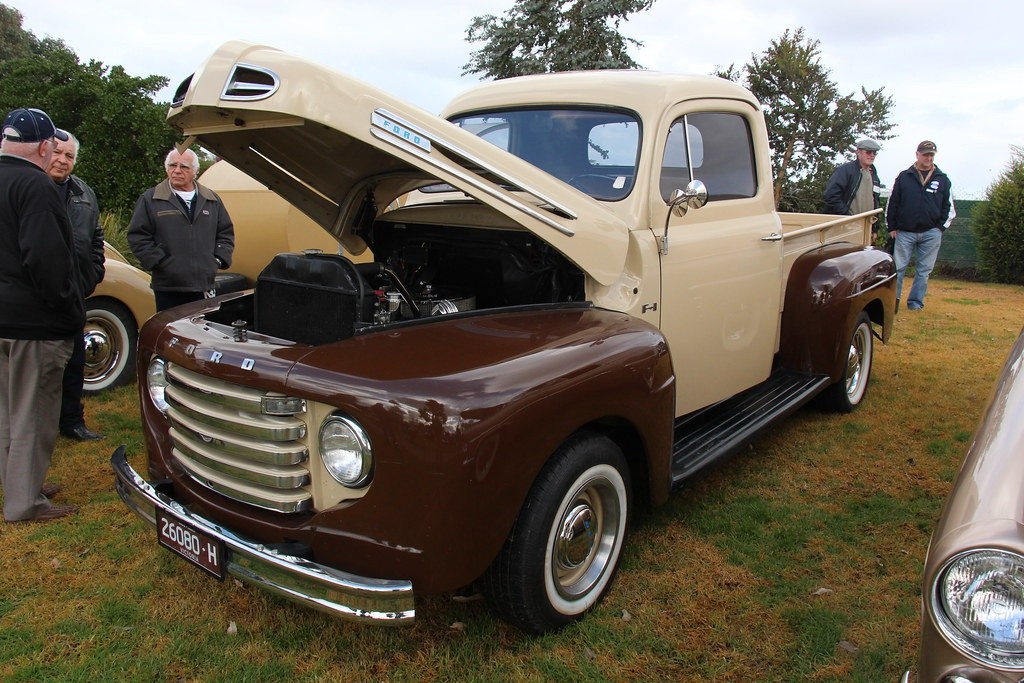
[109,40,898,637]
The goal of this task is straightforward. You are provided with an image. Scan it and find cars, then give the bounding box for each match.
[896,321,1024,682]
[74,239,156,396]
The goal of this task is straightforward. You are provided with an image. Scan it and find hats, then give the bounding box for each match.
[857,139,881,150]
[1,107,69,142]
[917,141,937,155]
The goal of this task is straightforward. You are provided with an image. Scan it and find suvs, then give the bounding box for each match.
[194,160,498,302]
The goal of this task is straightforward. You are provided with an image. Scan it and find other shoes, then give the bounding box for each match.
[4,506,79,524]
[41,483,62,499]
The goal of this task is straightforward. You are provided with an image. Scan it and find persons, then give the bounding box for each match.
[884,140,957,316]
[38,127,106,441]
[824,140,880,248]
[0,109,87,523]
[127,145,236,315]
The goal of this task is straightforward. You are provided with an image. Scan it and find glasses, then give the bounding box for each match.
[862,150,878,155]
[922,153,935,156]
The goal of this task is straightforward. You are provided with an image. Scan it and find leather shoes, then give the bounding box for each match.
[60,423,107,442]
